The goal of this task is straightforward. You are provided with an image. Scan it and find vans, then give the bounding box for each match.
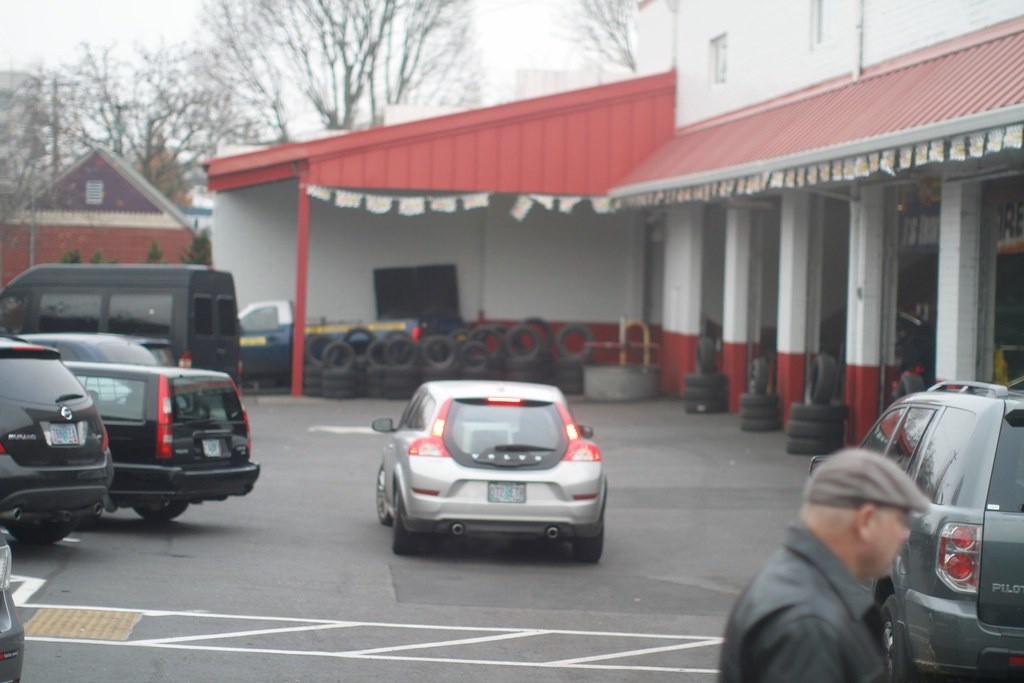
[2,260,244,396]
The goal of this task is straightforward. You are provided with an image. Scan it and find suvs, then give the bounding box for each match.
[807,379,1023,683]
[0,333,116,546]
[60,357,260,522]
[8,328,160,406]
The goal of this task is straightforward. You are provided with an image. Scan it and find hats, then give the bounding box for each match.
[804,448,931,513]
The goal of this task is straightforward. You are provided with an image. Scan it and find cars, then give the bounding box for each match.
[371,378,608,564]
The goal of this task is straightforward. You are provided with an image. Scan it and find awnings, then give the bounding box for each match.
[607,14,1024,212]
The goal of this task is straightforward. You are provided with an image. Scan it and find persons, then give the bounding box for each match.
[718,446,932,683]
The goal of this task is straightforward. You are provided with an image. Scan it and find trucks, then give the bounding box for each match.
[238,298,423,348]
